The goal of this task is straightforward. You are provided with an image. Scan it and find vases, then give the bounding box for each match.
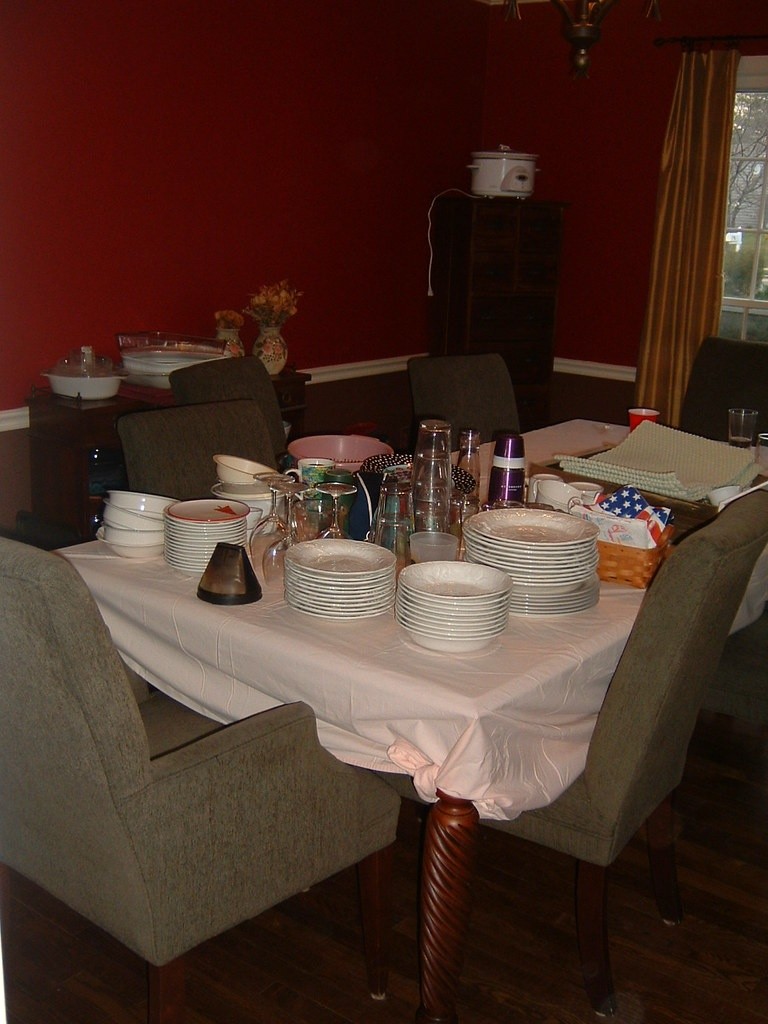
[251,326,289,376]
[216,328,246,357]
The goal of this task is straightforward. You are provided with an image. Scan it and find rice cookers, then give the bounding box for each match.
[467,144,544,200]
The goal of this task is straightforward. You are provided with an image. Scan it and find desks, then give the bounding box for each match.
[47,418,768,1024]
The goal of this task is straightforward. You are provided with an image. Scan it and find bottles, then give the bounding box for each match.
[373,476,411,591]
[452,479,464,556]
[409,419,451,534]
[456,429,480,550]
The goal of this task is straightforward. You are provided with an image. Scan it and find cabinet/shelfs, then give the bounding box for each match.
[426,193,573,432]
[23,371,312,542]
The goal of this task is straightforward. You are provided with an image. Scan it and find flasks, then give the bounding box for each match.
[487,434,525,509]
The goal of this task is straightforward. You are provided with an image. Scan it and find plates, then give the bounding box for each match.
[163,500,251,572]
[283,508,600,653]
[360,453,475,495]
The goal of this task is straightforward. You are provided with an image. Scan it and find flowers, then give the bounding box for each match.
[214,309,245,329]
[241,277,305,328]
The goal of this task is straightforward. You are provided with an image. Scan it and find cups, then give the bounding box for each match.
[247,507,263,543]
[708,486,742,506]
[525,474,604,514]
[628,409,661,433]
[284,458,335,500]
[409,532,459,566]
[757,434,768,477]
[727,408,758,451]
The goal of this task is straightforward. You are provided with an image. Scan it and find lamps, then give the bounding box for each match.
[499,0,663,80]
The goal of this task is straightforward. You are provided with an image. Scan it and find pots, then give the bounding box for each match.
[39,368,130,399]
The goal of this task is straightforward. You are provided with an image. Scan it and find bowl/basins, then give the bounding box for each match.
[94,490,180,559]
[211,454,286,519]
[120,346,232,389]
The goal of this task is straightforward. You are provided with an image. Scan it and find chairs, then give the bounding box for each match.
[679,336,768,446]
[0,536,402,1024]
[116,399,277,500]
[406,352,521,453]
[168,353,288,461]
[374,488,768,1018]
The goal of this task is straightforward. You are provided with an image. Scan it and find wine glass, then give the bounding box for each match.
[263,482,310,589]
[249,472,295,582]
[314,481,358,540]
